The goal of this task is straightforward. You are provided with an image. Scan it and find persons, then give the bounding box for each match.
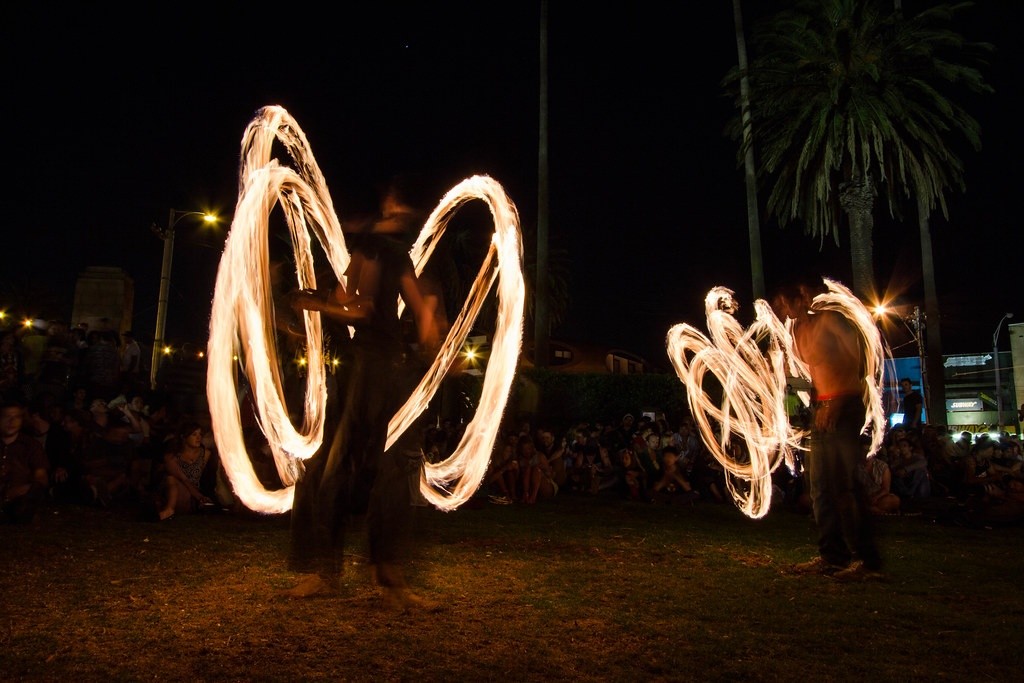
[877,426,1024,520]
[428,413,801,506]
[0,303,283,526]
[900,378,923,429]
[284,179,456,596]
[785,382,803,417]
[782,270,890,582]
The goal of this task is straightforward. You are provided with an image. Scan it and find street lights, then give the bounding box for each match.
[906,306,932,425]
[990,311,1013,432]
[149,206,221,395]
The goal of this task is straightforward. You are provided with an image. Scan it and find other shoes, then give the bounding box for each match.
[150,509,176,522]
[374,576,445,612]
[280,572,339,598]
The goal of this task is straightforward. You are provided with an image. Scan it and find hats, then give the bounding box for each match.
[623,413,635,422]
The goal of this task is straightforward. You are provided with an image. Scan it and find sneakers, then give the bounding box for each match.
[793,557,842,574]
[834,560,884,581]
